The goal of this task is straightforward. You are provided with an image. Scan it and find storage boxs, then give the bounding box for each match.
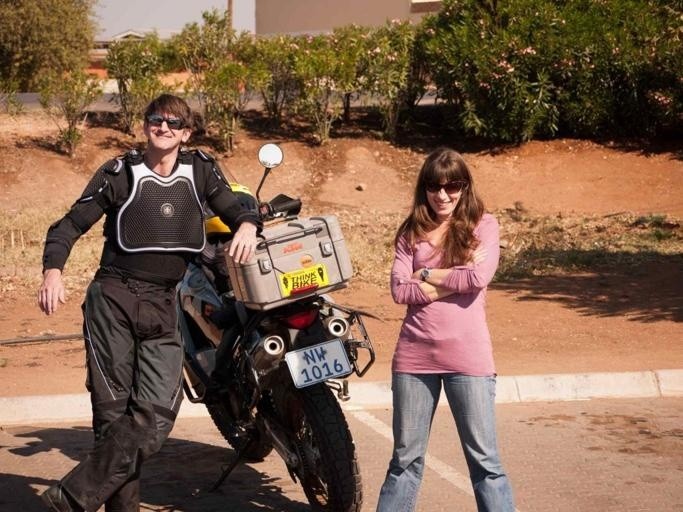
[223,215,353,305]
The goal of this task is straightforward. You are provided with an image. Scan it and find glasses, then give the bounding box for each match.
[426,181,465,194]
[146,114,188,130]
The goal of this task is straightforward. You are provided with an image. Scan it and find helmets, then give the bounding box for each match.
[202,182,255,243]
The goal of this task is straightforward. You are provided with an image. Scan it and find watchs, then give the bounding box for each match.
[419,267,431,281]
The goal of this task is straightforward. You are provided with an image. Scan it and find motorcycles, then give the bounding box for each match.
[181,142,375,509]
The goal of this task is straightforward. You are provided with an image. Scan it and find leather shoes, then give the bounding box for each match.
[41,484,73,511]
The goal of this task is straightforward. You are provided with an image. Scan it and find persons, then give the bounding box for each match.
[375,147,517,512]
[35,94,264,512]
